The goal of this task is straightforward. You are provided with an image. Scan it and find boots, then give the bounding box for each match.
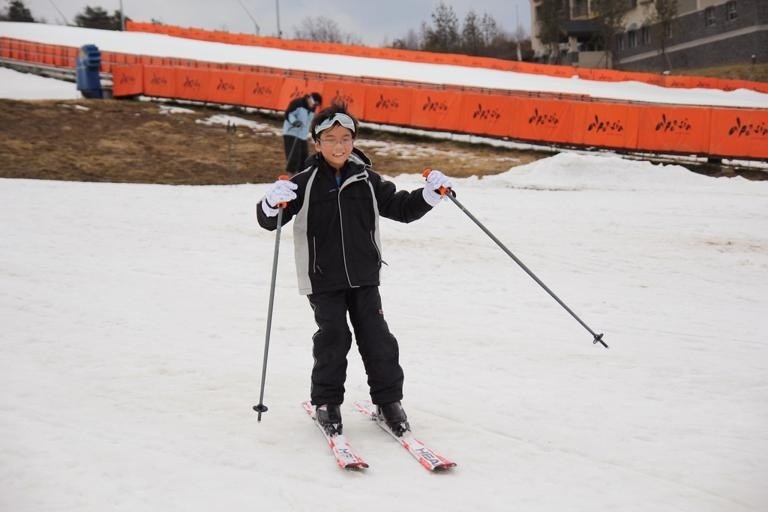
[377,401,411,438]
[316,405,342,436]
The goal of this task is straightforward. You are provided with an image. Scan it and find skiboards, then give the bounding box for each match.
[301,399,457,471]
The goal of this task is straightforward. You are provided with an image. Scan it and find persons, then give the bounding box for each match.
[257,103,452,437]
[283,93,322,176]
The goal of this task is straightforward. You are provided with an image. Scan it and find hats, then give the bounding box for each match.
[310,93,321,106]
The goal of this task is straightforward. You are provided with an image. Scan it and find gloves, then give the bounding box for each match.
[262,180,298,218]
[422,170,451,207]
[293,121,302,128]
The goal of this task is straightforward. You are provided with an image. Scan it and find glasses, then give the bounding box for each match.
[315,113,355,135]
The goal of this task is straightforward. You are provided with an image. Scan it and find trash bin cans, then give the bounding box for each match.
[76,44,101,98]
